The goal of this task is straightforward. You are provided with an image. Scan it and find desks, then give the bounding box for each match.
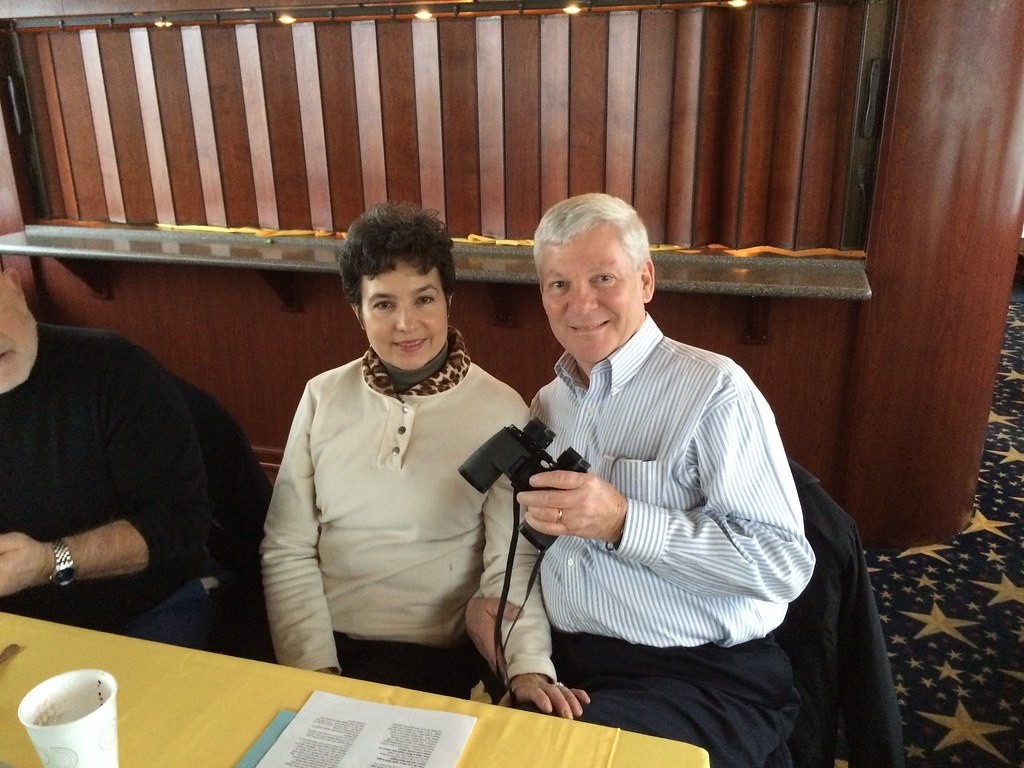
[0,609,712,768]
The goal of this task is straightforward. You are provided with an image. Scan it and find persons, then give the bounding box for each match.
[0,257,226,651]
[478,191,816,768]
[260,201,592,720]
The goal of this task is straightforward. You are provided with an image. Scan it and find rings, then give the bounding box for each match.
[554,682,564,688]
[556,508,563,522]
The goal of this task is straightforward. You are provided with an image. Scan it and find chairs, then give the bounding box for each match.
[756,452,864,768]
[163,375,274,659]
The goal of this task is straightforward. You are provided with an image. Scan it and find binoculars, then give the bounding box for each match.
[456,417,592,553]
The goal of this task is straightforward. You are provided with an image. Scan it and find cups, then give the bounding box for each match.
[18,669,119,768]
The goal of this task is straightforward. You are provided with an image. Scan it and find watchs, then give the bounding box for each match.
[48,538,77,587]
